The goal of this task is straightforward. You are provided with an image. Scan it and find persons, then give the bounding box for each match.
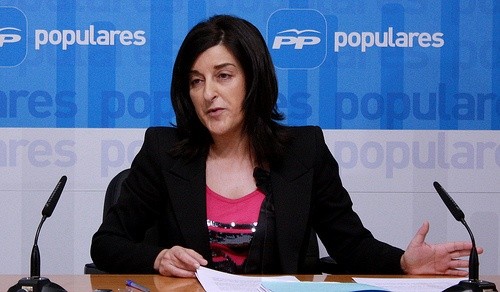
[89,14,483,280]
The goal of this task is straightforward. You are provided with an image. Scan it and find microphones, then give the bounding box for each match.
[8,175,67,292]
[434,182,498,292]
[253,168,268,177]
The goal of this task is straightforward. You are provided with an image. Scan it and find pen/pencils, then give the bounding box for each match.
[126,280,150,292]
